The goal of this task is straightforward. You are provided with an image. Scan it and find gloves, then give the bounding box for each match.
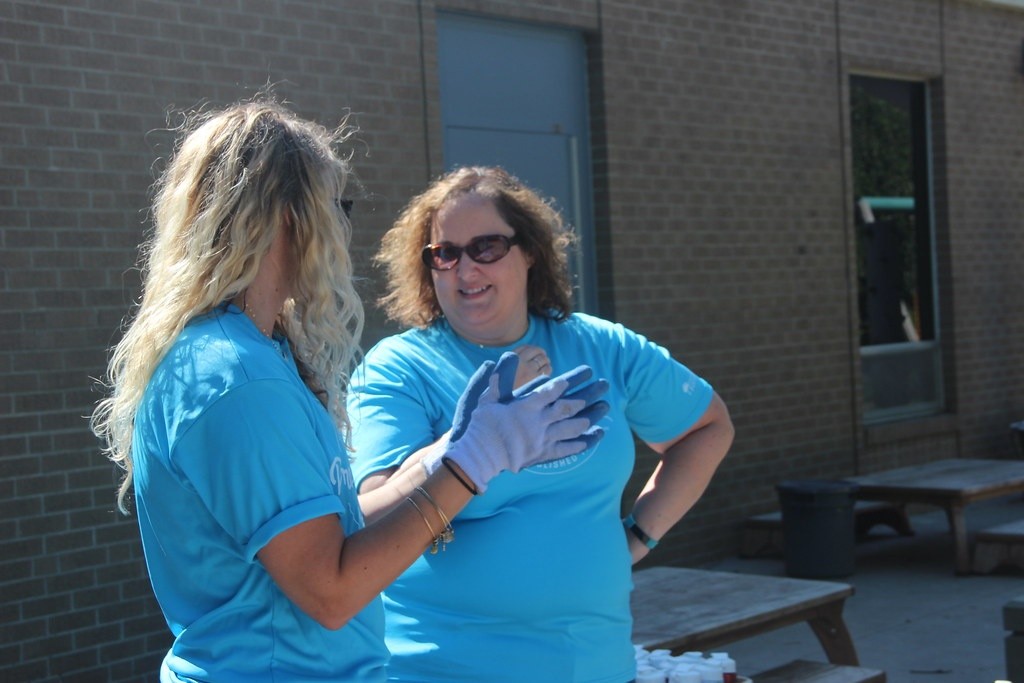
[420,351,610,496]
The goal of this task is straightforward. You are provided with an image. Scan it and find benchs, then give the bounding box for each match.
[753,502,878,549]
[748,660,887,683]
[966,510,1024,572]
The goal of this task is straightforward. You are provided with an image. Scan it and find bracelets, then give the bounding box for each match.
[441,457,479,496]
[407,486,454,554]
[623,513,658,549]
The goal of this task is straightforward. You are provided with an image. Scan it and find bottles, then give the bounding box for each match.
[632,642,738,683]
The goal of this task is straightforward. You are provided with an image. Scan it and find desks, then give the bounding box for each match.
[841,459,1023,576]
[631,566,859,656]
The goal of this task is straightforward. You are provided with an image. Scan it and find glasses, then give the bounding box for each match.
[333,198,353,219]
[422,234,521,271]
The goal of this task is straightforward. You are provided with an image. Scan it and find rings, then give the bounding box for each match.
[533,359,542,370]
[541,370,544,375]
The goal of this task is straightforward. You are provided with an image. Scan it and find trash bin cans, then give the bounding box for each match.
[772,478,866,579]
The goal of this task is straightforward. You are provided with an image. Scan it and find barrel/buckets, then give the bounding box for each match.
[775,479,856,582]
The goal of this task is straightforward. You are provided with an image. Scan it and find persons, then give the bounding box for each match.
[344,166,735,683]
[95,99,608,683]
